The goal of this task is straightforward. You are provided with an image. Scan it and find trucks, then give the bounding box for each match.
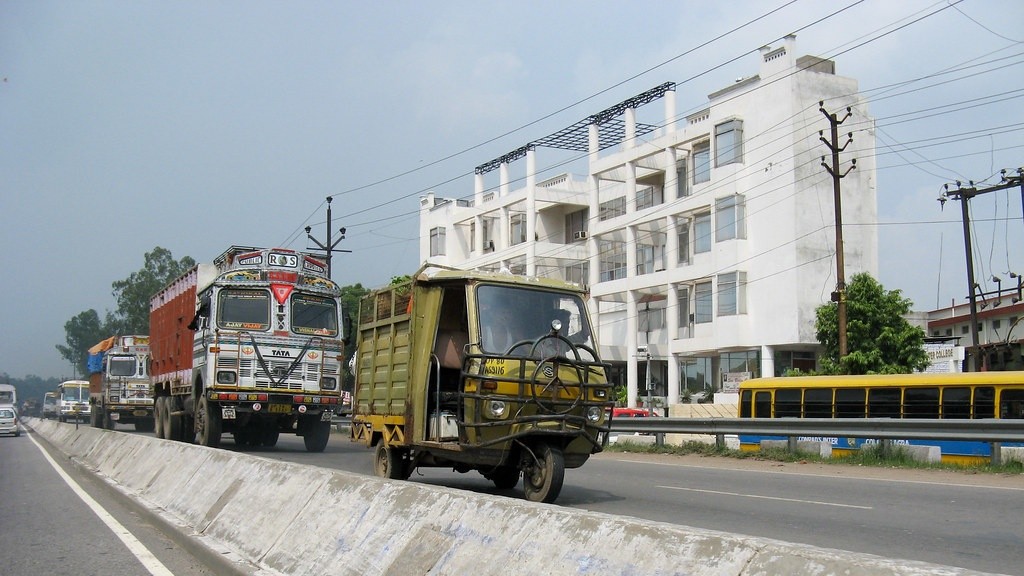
[147,244,346,452]
[86,335,157,432]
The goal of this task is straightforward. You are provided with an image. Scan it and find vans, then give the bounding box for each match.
[607,407,662,420]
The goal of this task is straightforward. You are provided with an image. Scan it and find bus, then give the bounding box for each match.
[42,392,58,418]
[0,384,19,417]
[734,370,1024,470]
[55,380,93,424]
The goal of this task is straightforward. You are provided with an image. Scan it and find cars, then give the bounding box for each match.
[0,407,21,437]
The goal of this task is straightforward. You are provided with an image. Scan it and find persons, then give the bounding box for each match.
[481,294,539,359]
[528,296,590,360]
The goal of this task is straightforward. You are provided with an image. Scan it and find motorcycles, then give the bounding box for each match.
[345,263,615,504]
